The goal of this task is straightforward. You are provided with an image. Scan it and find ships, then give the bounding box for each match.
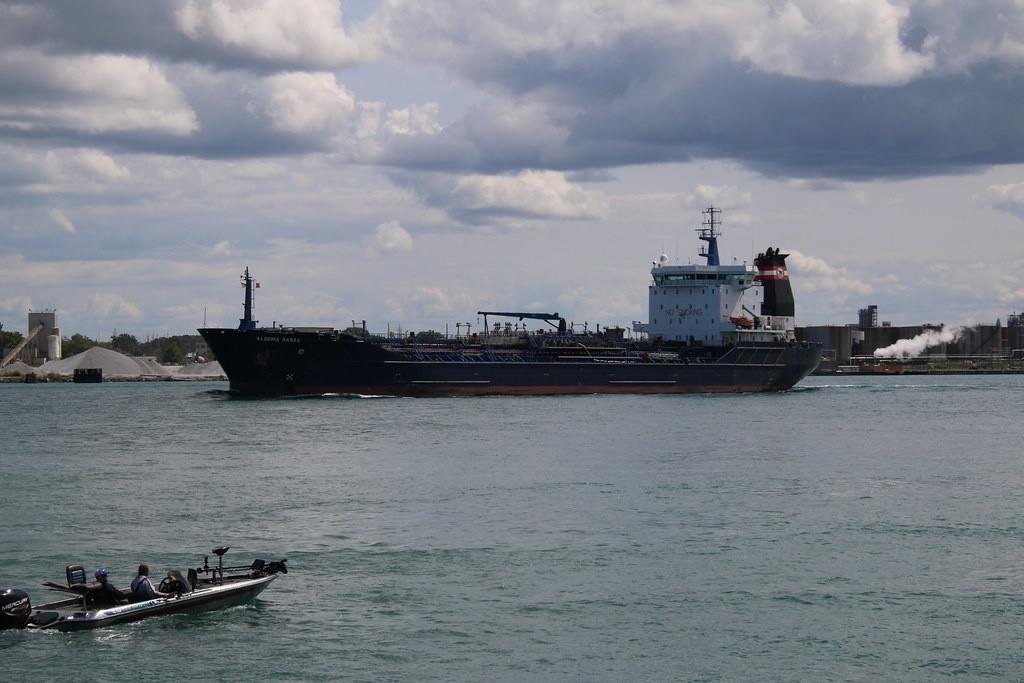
[198,204,826,395]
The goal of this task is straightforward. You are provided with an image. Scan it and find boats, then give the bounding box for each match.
[0,545,289,632]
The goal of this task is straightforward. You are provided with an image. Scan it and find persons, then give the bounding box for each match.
[95,568,126,605]
[131,564,175,600]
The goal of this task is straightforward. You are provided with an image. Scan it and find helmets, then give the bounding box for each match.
[95,568,110,576]
